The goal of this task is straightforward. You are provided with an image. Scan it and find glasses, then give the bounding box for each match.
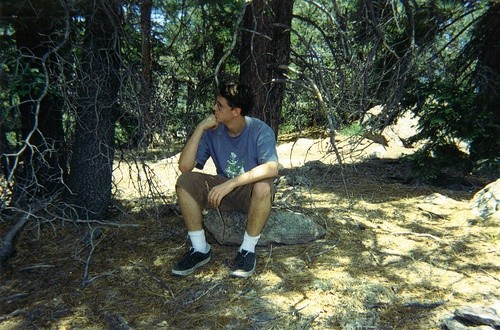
[215,100,236,110]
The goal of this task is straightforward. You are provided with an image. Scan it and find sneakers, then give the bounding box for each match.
[172,243,211,275]
[229,248,256,278]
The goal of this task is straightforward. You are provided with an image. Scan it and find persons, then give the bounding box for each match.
[170,83,281,279]
